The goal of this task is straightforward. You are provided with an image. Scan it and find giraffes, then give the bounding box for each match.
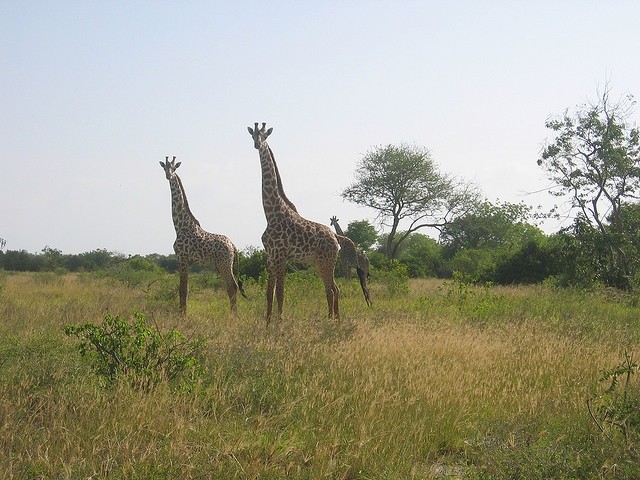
[159,156,247,316]
[330,216,369,281]
[248,123,374,324]
